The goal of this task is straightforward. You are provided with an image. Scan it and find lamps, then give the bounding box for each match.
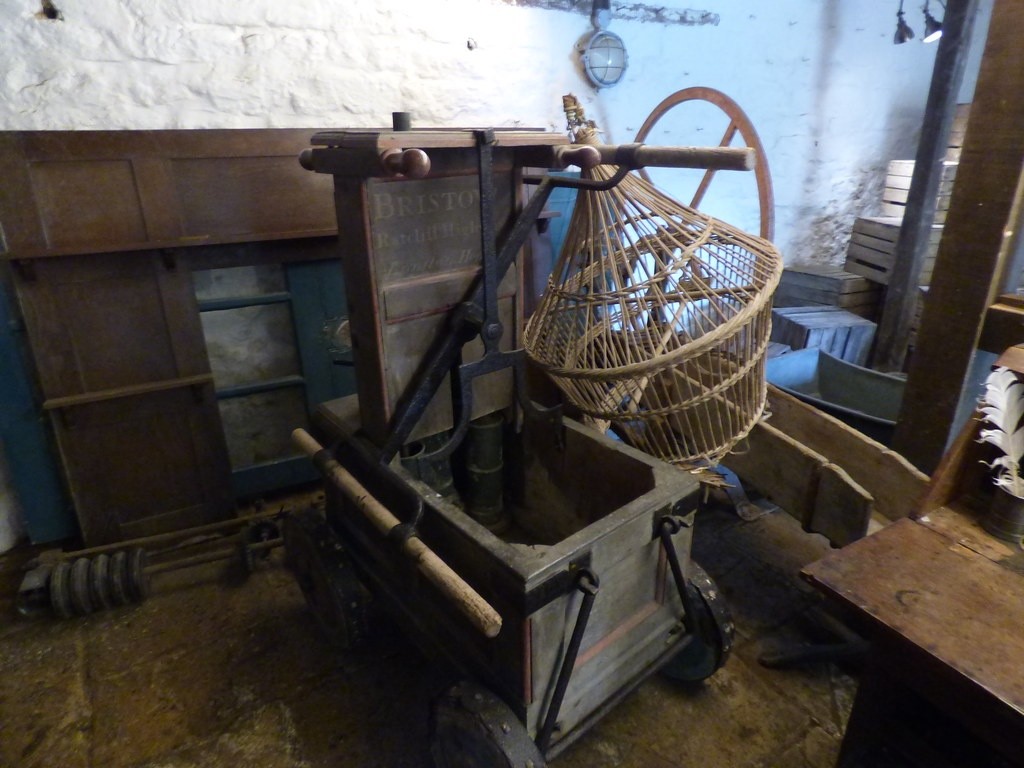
[923,0,945,44]
[578,0,628,88]
[894,1,914,44]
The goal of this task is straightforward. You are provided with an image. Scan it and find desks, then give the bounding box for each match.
[761,497,1024,768]
